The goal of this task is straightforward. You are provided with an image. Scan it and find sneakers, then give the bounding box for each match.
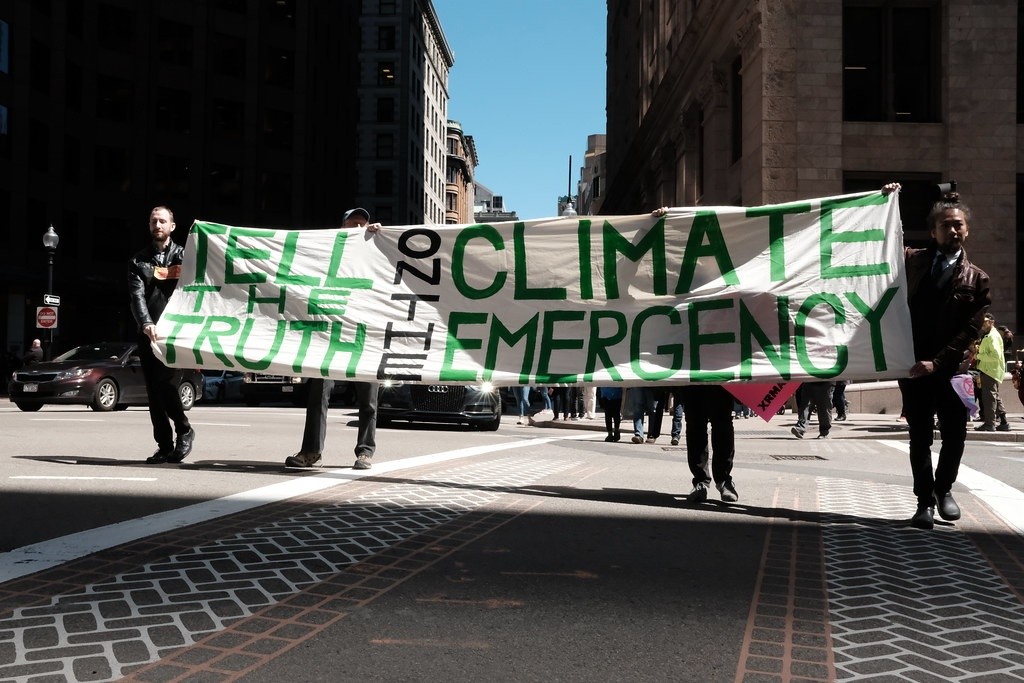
[285,449,322,467]
[687,484,709,501]
[720,481,738,502]
[353,451,372,469]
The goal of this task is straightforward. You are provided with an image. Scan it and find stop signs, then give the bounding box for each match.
[38,308,56,327]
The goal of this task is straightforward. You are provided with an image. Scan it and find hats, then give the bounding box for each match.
[342,208,370,226]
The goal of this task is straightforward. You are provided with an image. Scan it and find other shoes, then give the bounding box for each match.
[791,425,804,439]
[974,424,996,431]
[631,436,643,443]
[834,416,845,421]
[671,436,678,446]
[817,431,829,438]
[646,436,656,443]
[996,425,1011,431]
[605,431,621,442]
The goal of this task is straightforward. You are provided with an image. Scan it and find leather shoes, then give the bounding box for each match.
[170,428,195,463]
[933,485,961,520]
[911,505,935,528]
[146,447,176,464]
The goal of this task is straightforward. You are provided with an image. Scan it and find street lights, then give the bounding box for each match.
[43,223,60,344]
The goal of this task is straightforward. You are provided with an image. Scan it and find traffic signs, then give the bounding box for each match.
[44,294,61,306]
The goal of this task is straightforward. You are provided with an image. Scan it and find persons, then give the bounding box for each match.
[285,208,382,469]
[882,182,992,530]
[896,313,1024,431]
[651,206,740,505]
[791,380,852,441]
[127,206,195,465]
[500,386,787,445]
[0,336,51,402]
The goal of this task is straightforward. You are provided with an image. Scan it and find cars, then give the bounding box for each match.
[376,383,502,432]
[200,368,246,407]
[240,372,357,407]
[8,343,205,412]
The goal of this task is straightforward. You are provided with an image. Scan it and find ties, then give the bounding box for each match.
[931,254,946,282]
